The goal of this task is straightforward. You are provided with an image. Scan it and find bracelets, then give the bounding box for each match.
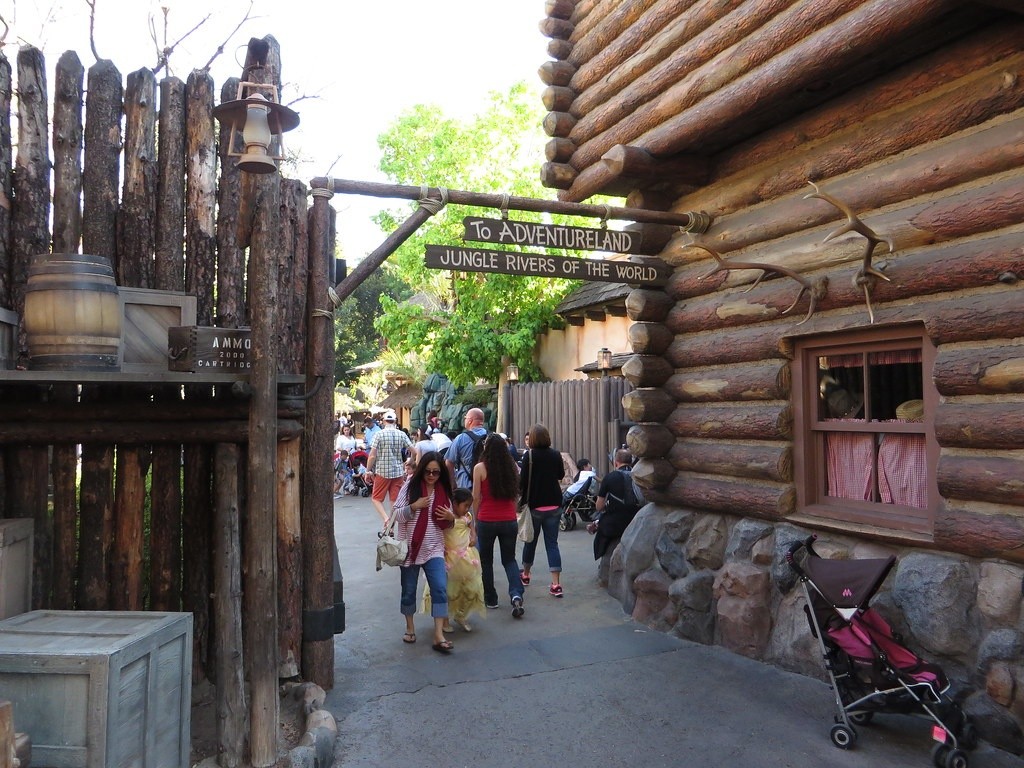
[366,471,371,474]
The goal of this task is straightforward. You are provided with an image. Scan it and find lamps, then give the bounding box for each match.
[506,362,519,385]
[211,55,300,174]
[597,348,612,377]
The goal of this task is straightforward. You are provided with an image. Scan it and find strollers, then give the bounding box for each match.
[348,447,375,497]
[785,534,978,768]
[560,476,599,531]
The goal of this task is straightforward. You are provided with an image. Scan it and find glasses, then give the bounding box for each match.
[464,416,468,420]
[423,469,441,477]
[482,440,486,446]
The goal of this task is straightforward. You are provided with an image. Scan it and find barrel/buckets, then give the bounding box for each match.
[24,253,123,373]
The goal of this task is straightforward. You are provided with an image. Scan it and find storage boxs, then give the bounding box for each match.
[0,609,193,768]
[167,326,252,372]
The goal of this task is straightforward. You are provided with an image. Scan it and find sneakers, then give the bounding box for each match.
[519,569,530,585]
[549,583,564,597]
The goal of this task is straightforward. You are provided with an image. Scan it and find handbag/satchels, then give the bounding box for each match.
[376,507,409,570]
[517,502,534,543]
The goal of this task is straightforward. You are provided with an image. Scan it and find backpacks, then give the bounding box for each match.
[610,463,648,512]
[459,429,493,484]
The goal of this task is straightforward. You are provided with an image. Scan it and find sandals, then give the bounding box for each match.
[378,529,394,539]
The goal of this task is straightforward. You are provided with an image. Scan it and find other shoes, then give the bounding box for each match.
[334,491,343,499]
[586,521,598,536]
[453,614,472,632]
[511,595,525,618]
[345,489,350,495]
[485,602,499,608]
[442,621,455,633]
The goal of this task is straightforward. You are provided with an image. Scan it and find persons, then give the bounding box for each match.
[500,433,519,463]
[517,423,565,596]
[564,459,603,498]
[402,411,453,479]
[347,415,354,434]
[419,488,487,632]
[334,449,373,497]
[471,433,525,616]
[336,425,356,453]
[392,451,456,651]
[444,407,488,551]
[586,449,638,561]
[365,412,416,538]
[522,432,530,458]
[364,418,383,454]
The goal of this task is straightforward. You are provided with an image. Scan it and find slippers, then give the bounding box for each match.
[403,632,416,643]
[432,638,454,650]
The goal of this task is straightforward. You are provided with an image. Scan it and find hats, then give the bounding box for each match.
[384,412,398,421]
[364,416,375,425]
[418,424,427,434]
